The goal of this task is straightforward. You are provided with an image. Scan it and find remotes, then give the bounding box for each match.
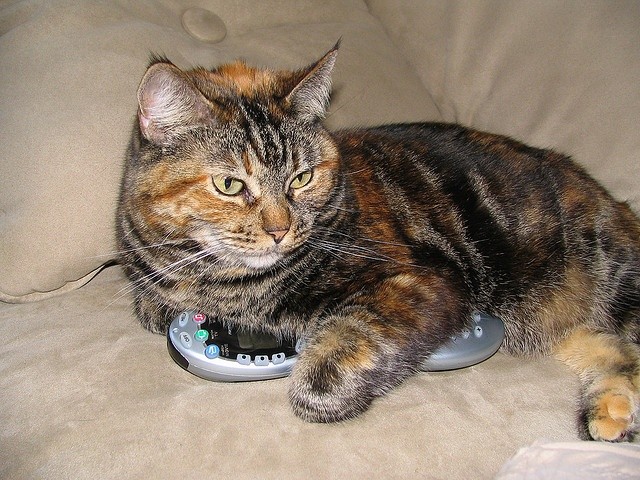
[167,306,506,382]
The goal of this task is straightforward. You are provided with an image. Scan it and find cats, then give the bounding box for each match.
[112,32,640,443]
